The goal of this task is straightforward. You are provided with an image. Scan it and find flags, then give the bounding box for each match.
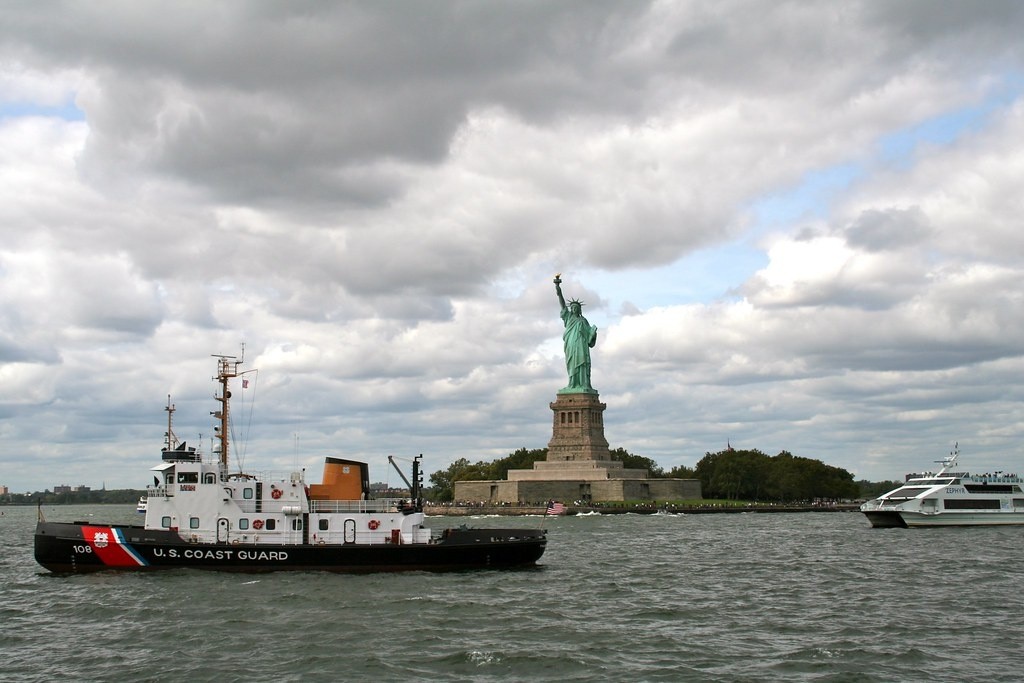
[242,380,249,388]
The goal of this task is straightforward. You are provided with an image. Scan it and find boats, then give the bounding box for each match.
[859,443,1024,528]
[35,341,548,575]
[137,495,147,513]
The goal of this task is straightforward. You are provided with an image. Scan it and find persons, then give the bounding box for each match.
[425,497,868,510]
[925,470,1017,478]
[397,498,423,511]
[556,283,597,390]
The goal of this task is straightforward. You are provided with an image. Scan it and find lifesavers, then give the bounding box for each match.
[253,519,263,529]
[271,490,281,499]
[368,520,378,529]
